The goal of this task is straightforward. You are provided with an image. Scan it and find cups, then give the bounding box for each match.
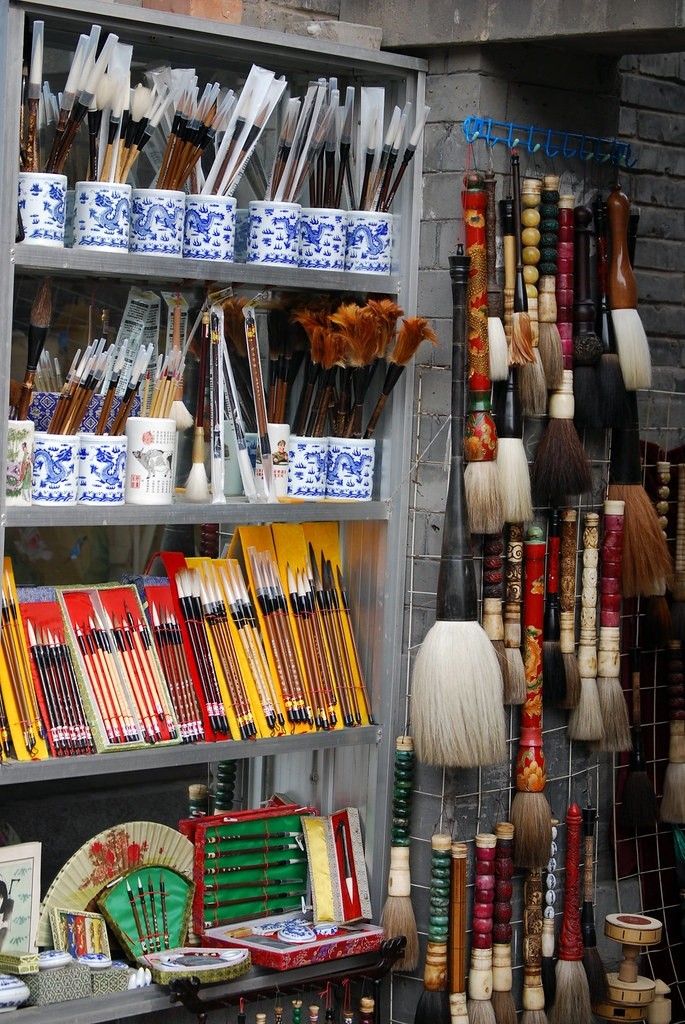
[5,419,35,508]
[33,434,81,506]
[17,172,68,248]
[220,419,247,496]
[299,208,346,273]
[245,433,258,474]
[64,190,75,248]
[125,417,177,504]
[248,200,302,269]
[346,210,394,276]
[325,437,376,503]
[254,423,290,497]
[288,435,329,501]
[73,181,132,254]
[235,208,250,263]
[131,189,185,258]
[182,194,237,264]
[76,432,129,506]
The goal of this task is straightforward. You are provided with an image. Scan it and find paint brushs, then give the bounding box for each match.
[21,22,431,213]
[126,872,170,953]
[203,831,307,928]
[339,822,354,905]
[380,150,685,1024]
[175,540,376,739]
[1,567,204,766]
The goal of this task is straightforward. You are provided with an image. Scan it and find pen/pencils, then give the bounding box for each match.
[8,277,439,505]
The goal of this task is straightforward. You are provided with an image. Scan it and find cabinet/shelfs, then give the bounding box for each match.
[0,0,430,1024]
